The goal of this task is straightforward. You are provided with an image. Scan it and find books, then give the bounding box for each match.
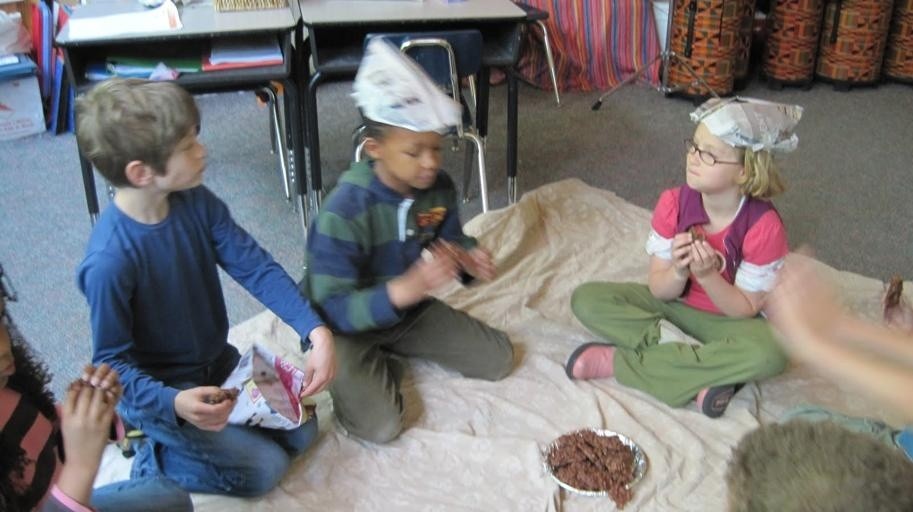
[84,29,283,80]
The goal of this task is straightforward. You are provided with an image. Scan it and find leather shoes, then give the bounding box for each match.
[566,343,615,380]
[695,385,735,417]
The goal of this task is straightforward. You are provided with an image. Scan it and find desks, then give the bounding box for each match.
[54,0,301,241]
[299,0,548,219]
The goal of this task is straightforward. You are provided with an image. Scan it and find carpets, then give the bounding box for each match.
[92,176,913,511]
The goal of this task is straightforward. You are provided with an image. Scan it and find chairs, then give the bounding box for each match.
[353,33,490,225]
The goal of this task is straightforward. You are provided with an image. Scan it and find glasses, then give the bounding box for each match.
[684,139,739,166]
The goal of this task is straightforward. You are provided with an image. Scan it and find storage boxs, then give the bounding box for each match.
[1,75,47,145]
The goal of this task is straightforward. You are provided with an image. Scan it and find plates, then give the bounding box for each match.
[540,427,650,498]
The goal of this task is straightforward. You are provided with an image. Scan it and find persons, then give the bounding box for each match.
[562,90,803,417]
[69,70,339,498]
[0,270,191,512]
[763,241,913,451]
[298,34,515,444]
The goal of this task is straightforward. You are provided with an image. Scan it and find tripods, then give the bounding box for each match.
[592,0,722,111]
